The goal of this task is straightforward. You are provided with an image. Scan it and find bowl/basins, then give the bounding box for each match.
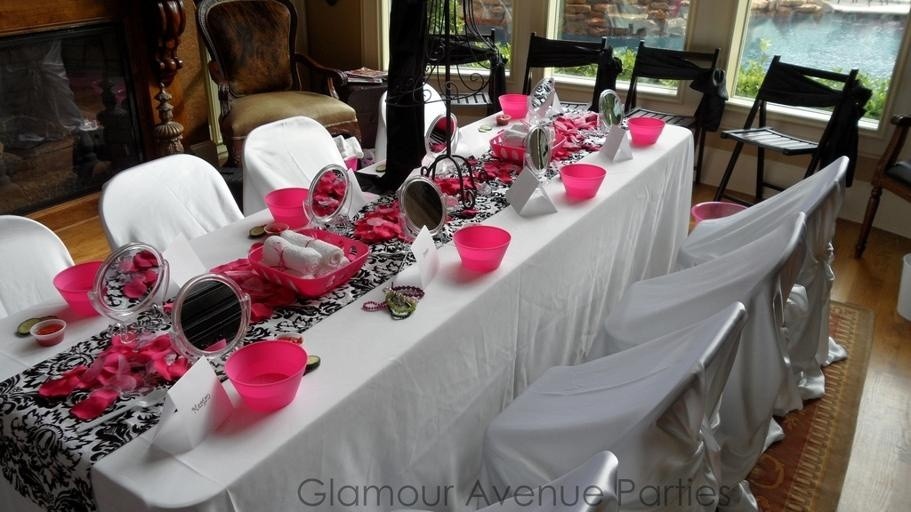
[561,162,607,201]
[53,260,116,319]
[264,186,314,230]
[452,224,513,274]
[32,319,66,347]
[499,93,531,120]
[627,115,667,146]
[693,200,751,226]
[226,341,310,412]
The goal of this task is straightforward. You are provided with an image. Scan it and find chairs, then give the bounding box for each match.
[0,0,911,511]
